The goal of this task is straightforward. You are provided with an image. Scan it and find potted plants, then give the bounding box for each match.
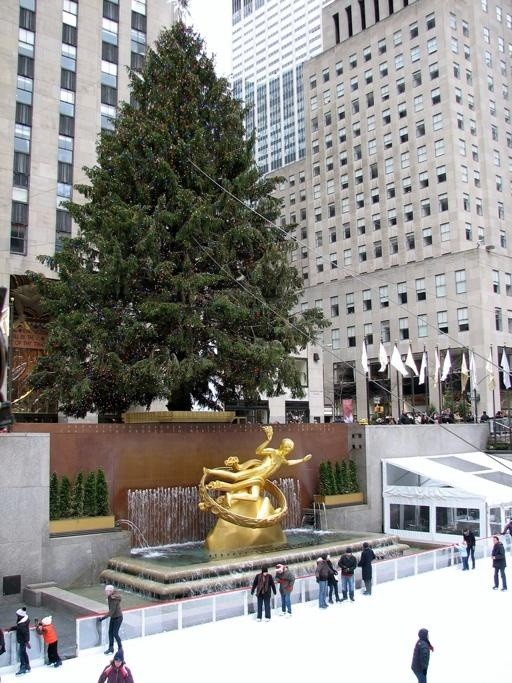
[49,465,115,534]
[313,458,363,504]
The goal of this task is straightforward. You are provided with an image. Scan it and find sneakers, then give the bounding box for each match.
[16,666,30,674]
[47,660,61,667]
[105,649,113,654]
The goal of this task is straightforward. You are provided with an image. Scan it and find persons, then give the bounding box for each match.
[251,567,276,621]
[500,517,512,537]
[490,536,507,590]
[453,540,469,571]
[99,585,123,654]
[36,615,62,667]
[0,629,6,656]
[463,528,476,569]
[202,426,313,508]
[98,650,134,683]
[314,542,376,608]
[5,606,31,674]
[370,404,506,423]
[411,628,433,683]
[276,564,295,617]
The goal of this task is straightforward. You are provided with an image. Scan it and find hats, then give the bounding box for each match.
[42,616,53,626]
[15,607,26,618]
[105,585,115,593]
[114,650,124,660]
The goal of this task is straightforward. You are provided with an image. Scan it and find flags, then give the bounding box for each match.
[361,341,512,390]
